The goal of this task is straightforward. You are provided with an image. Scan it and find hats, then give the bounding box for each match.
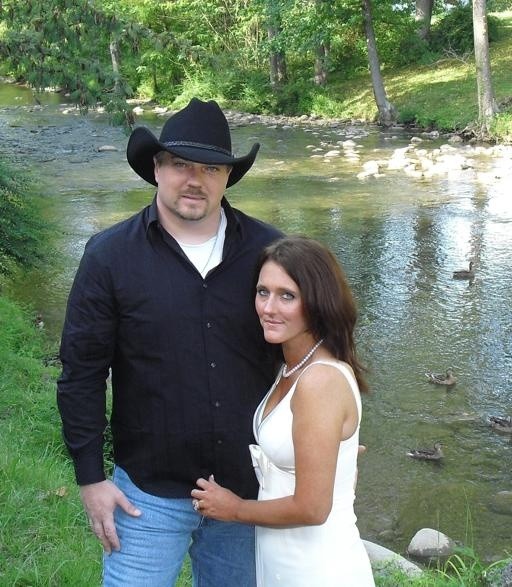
[125,96,260,188]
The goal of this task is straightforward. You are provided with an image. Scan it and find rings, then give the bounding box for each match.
[192,500,200,512]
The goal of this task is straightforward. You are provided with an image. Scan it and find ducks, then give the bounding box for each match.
[425,367,456,385]
[407,440,446,460]
[453,261,474,280]
[487,415,512,434]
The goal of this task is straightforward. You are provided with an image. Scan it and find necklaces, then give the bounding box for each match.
[199,213,222,274]
[282,339,323,378]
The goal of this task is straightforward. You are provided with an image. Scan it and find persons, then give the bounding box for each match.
[57,97,289,587]
[191,235,376,587]
